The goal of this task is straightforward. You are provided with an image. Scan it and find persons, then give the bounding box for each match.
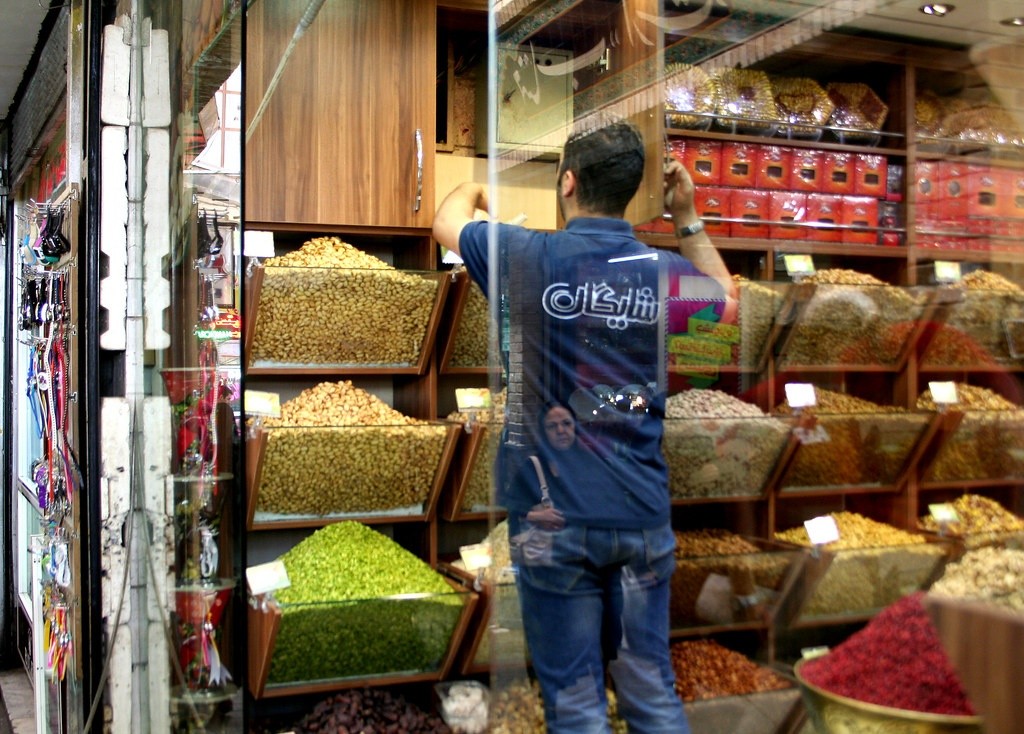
[433,109,738,734]
[215,385,238,436]
[505,400,616,576]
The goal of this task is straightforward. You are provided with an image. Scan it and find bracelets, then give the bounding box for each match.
[676,221,705,239]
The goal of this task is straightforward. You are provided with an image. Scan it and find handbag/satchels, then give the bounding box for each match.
[509,497,557,567]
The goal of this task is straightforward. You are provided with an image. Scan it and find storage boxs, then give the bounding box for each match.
[238,138,1024,734]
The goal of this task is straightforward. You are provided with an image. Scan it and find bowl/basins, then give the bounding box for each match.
[793,653,984,733]
